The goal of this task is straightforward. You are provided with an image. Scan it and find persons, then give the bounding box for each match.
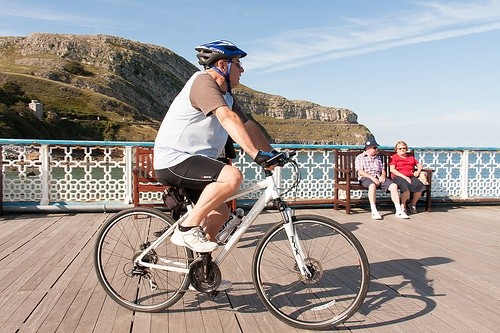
[153,39,289,291]
[355,139,410,219]
[389,141,424,215]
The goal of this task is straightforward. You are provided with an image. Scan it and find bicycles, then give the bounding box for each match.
[93,149,371,331]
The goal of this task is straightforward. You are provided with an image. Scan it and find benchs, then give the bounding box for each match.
[132,146,237,218]
[334,149,435,214]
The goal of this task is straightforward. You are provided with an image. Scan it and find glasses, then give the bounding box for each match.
[373,146,378,149]
[225,60,243,66]
[396,148,406,150]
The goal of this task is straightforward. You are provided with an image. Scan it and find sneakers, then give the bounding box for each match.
[170,224,218,253]
[188,278,233,291]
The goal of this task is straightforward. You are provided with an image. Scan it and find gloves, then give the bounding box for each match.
[254,150,280,170]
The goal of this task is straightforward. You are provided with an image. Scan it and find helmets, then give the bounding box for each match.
[195,39,247,68]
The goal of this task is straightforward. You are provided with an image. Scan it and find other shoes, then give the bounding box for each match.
[396,210,409,219]
[408,204,417,213]
[403,209,408,214]
[372,212,382,219]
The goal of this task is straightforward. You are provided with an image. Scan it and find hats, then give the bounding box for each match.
[363,139,380,150]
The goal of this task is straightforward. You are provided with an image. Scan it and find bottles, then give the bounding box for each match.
[218,208,244,242]
